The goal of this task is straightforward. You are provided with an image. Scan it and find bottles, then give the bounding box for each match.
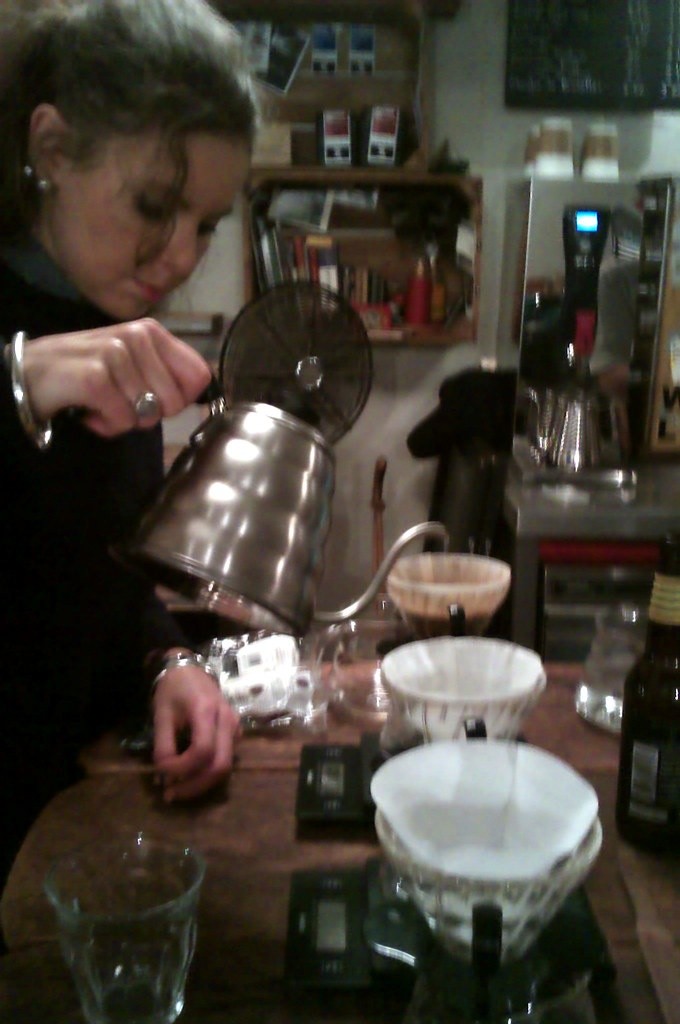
[616,533,680,858]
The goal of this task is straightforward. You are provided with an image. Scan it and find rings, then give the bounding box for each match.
[132,388,158,416]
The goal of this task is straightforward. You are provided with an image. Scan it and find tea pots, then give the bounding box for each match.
[67,283,449,636]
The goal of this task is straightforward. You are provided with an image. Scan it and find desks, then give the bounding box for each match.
[1,656,680,1024]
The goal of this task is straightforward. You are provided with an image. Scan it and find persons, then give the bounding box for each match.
[0,0,242,894]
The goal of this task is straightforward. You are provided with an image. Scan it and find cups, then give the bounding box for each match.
[378,636,546,748]
[575,606,647,731]
[582,124,620,181]
[523,119,574,180]
[386,553,511,641]
[41,832,204,1024]
[370,741,605,964]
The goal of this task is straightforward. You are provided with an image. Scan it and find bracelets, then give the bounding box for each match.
[151,650,222,690]
[10,329,52,451]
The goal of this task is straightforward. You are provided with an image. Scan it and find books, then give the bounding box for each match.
[255,217,392,337]
